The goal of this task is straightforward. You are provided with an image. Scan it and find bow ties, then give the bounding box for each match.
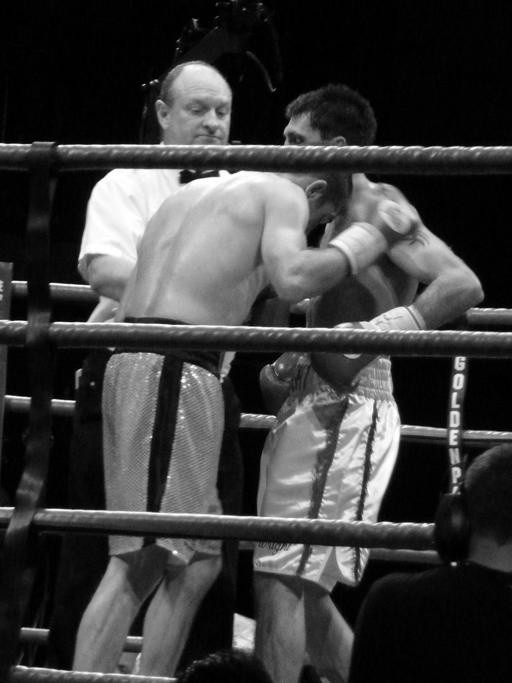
[180,170,219,184]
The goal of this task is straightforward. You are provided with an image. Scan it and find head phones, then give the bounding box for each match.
[432,484,472,567]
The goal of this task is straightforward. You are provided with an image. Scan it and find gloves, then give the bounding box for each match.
[366,197,419,247]
[260,357,297,395]
[308,321,380,389]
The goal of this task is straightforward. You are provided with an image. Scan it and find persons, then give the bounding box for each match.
[247,82,488,682]
[66,139,424,682]
[344,441,512,682]
[176,648,272,683]
[43,59,237,680]
